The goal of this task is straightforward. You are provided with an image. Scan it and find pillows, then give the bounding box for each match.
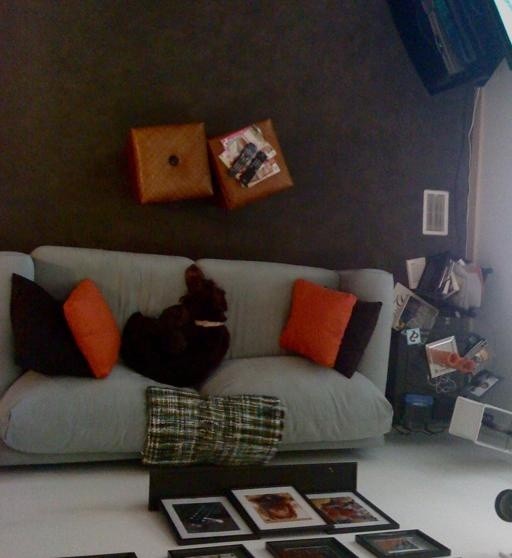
[12,273,121,379]
[280,278,381,380]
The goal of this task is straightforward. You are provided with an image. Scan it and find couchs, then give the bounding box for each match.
[1,245,396,465]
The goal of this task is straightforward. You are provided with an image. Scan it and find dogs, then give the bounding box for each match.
[118,263,231,392]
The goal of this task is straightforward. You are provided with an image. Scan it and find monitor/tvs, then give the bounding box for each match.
[385,0,512,96]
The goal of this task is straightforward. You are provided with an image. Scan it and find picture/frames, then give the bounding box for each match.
[158,485,452,557]
